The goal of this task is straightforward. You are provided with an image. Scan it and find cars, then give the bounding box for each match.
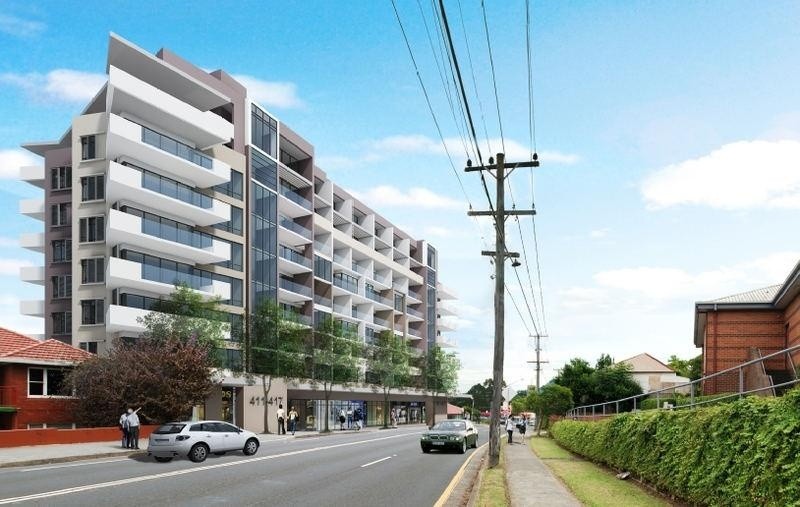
[421,418,481,455]
[500,414,534,427]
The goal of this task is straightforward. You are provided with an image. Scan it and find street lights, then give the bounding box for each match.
[481,250,522,470]
[505,377,525,402]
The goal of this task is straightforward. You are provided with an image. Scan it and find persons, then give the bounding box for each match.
[126,407,141,449]
[346,406,354,429]
[275,403,287,435]
[119,408,131,448]
[518,413,527,444]
[353,407,363,430]
[287,405,300,435]
[505,412,516,444]
[338,406,347,430]
[389,407,398,427]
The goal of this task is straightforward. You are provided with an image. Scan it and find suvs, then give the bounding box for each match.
[147,419,262,465]
